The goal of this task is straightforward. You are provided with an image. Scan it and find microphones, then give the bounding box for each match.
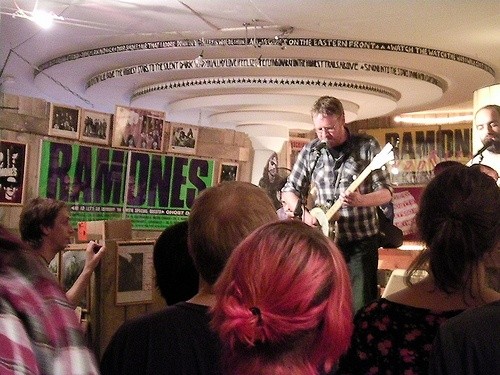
[310,141,327,152]
[474,138,494,158]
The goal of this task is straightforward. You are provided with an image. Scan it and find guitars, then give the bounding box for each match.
[309,142,394,246]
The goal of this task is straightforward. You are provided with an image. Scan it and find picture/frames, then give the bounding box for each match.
[116,242,156,306]
[48,102,82,140]
[0,139,30,207]
[79,108,113,147]
[59,244,89,313]
[217,163,239,185]
[168,122,199,155]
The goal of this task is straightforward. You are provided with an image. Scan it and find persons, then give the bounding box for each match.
[318,164,500,375]
[0,176,21,202]
[221,165,236,181]
[19,196,107,312]
[65,255,84,291]
[257,153,292,210]
[0,225,100,375]
[152,219,200,305]
[205,217,357,375]
[464,104,500,185]
[62,169,149,209]
[51,105,196,152]
[280,95,395,312]
[101,179,283,375]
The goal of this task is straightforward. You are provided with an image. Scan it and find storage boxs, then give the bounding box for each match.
[77,219,132,242]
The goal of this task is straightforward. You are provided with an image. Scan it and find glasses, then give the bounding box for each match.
[315,120,340,132]
[270,159,277,166]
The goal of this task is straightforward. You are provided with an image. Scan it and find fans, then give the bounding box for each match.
[258,167,291,210]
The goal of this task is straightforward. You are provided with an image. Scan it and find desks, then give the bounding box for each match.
[111,104,166,153]
